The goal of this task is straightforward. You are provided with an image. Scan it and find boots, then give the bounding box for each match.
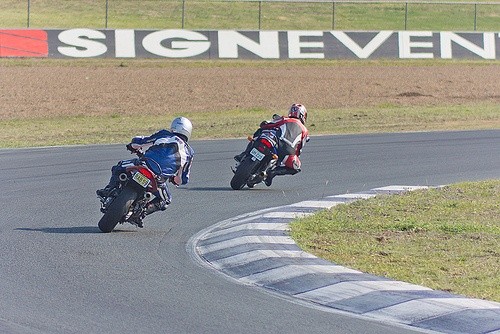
[96,170,121,196]
[234,141,252,161]
[130,198,165,229]
[262,165,300,187]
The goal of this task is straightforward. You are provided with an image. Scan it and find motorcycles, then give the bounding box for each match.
[95,144,183,233]
[230,114,316,190]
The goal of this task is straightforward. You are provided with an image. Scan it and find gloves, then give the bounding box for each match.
[169,175,179,186]
[126,143,137,153]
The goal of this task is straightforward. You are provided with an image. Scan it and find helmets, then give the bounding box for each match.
[171,117,192,142]
[289,103,307,124]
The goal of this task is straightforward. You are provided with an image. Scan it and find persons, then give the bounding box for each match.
[97,116,194,228]
[262,103,308,186]
